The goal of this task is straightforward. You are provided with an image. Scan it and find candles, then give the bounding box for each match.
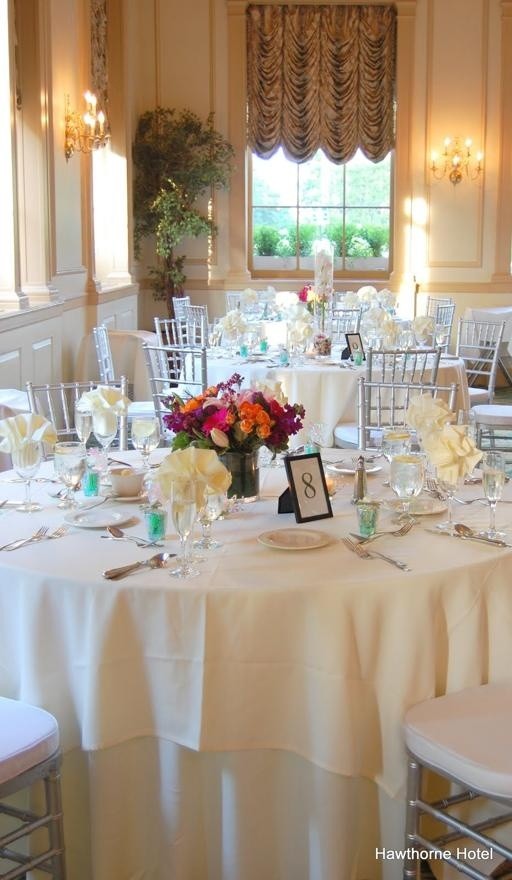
[429,136,483,170]
[84,90,107,135]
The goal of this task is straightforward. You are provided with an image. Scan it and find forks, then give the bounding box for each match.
[340,537,407,572]
[3,524,69,553]
[360,516,414,547]
[424,475,512,509]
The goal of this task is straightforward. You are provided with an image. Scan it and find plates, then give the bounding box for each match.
[326,462,379,474]
[257,527,336,552]
[66,510,138,530]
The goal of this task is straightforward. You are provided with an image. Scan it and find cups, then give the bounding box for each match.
[108,467,148,496]
[146,508,168,540]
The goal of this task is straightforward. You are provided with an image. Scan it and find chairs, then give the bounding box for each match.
[402,672,512,880]
[1,696,65,879]
[23,292,512,467]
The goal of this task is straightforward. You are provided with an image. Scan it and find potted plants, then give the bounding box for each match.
[131,106,240,368]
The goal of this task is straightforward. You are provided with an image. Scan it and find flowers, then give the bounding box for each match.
[342,286,394,313]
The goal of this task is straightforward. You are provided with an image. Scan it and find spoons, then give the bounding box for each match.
[107,529,161,551]
[456,523,504,547]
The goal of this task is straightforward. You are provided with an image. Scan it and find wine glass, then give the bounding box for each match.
[166,477,196,581]
[381,426,508,541]
[189,481,228,551]
[11,440,42,518]
[233,296,417,366]
[55,400,159,509]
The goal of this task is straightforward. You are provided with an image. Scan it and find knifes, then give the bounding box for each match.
[100,551,176,579]
[421,528,504,547]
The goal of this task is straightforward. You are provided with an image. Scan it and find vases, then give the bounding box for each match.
[218,452,259,503]
